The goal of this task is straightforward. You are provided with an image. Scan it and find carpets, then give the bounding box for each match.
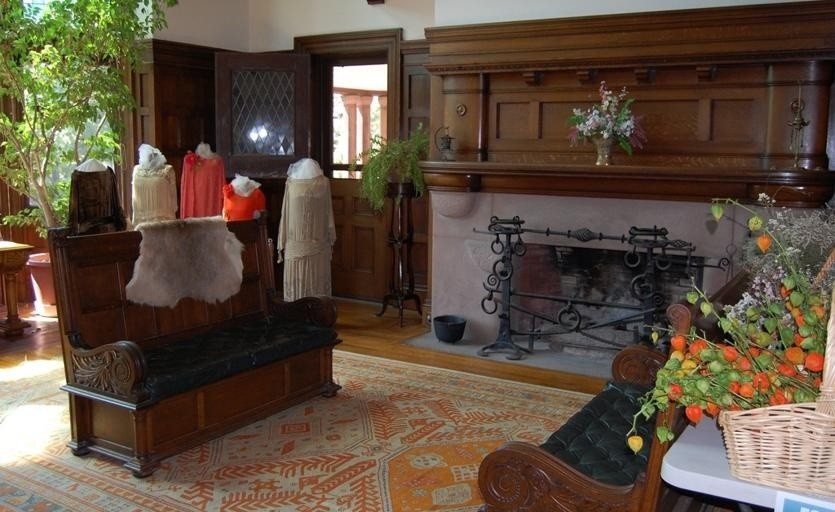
[0,349,597,511]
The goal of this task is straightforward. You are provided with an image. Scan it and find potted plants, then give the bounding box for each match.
[0,0,182,318]
[347,120,431,216]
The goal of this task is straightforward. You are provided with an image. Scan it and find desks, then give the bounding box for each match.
[0,239,34,338]
[660,412,835,512]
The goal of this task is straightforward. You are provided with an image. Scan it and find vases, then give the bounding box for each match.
[586,135,615,166]
[434,315,466,344]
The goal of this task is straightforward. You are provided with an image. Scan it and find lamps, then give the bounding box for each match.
[434,125,456,162]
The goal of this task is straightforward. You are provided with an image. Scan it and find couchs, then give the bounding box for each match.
[475,271,749,512]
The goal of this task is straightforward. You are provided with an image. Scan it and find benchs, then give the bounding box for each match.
[49,212,343,477]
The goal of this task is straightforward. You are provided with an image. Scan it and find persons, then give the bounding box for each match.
[179,142,225,219]
[130,143,179,225]
[276,159,336,301]
[222,175,268,222]
[67,158,128,235]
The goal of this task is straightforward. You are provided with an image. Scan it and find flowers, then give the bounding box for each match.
[567,77,649,155]
